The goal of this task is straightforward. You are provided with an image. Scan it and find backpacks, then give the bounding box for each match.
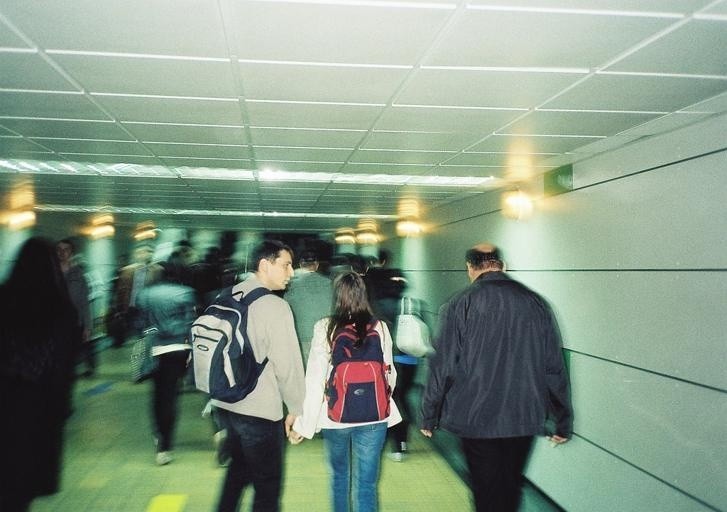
[324,317,391,423]
[190,287,273,403]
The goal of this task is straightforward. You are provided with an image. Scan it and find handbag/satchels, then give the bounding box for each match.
[396,315,436,357]
[130,327,159,384]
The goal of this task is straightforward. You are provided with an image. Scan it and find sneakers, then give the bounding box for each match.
[386,442,407,462]
[153,436,171,465]
[213,428,233,466]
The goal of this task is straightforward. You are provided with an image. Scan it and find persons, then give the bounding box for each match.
[213,238,306,511]
[289,272,405,512]
[0,233,84,510]
[419,243,575,510]
[52,238,89,424]
